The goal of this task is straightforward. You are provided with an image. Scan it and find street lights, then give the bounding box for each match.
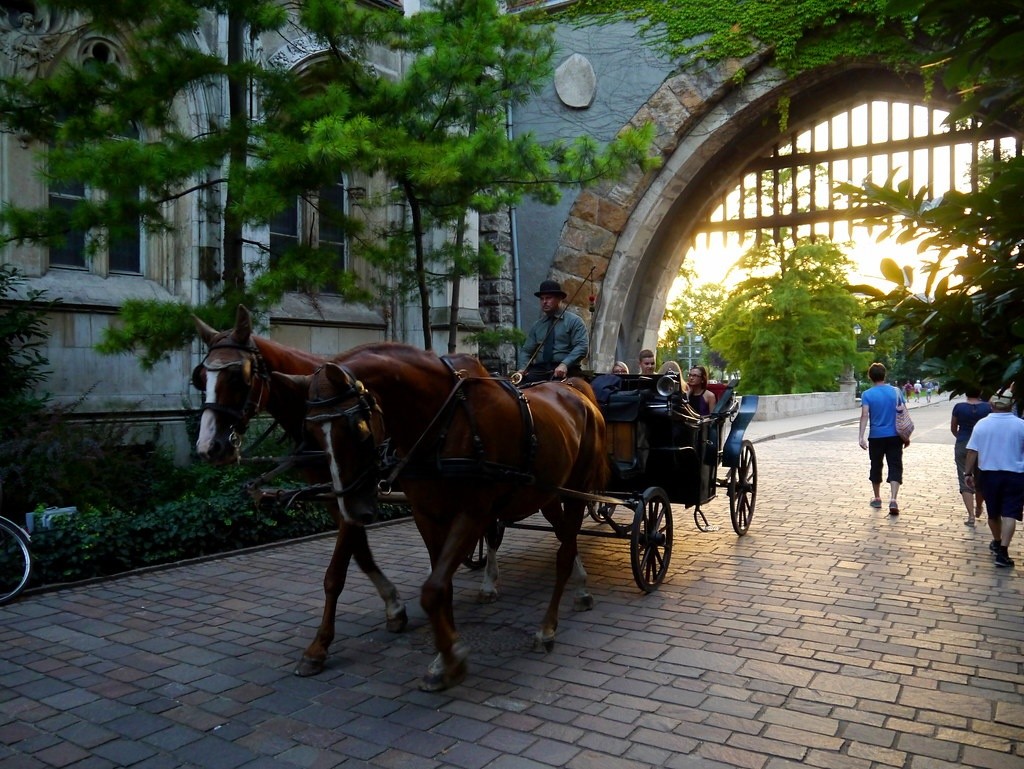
[676,321,704,380]
[852,322,878,398]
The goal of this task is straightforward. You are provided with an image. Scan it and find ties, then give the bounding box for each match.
[543,315,555,362]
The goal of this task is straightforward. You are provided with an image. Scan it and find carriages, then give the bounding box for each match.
[183,302,757,692]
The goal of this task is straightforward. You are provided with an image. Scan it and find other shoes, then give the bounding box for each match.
[989,541,1000,556]
[993,555,1015,568]
[870,499,882,509]
[963,519,977,528]
[975,506,984,518]
[887,500,901,515]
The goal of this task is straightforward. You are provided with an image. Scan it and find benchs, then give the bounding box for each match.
[688,381,732,465]
[585,373,654,422]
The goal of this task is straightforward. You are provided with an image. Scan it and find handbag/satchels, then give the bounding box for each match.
[894,386,915,442]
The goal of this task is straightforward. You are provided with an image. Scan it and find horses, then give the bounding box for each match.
[190,304,490,677]
[304,341,612,694]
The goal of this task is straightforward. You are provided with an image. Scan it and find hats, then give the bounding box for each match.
[990,389,1016,404]
[534,280,569,298]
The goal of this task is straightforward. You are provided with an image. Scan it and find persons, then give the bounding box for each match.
[688,365,716,416]
[914,380,922,403]
[612,361,629,374]
[858,363,910,513]
[950,381,992,527]
[904,380,912,403]
[519,281,589,384]
[1011,382,1024,420]
[638,349,656,374]
[924,382,934,403]
[658,361,690,397]
[964,389,1024,567]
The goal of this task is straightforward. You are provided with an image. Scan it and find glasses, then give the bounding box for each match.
[688,373,702,378]
[614,369,628,375]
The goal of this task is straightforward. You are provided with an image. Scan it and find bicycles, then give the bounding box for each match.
[0,513,37,606]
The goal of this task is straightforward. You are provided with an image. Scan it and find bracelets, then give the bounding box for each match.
[963,472,972,476]
